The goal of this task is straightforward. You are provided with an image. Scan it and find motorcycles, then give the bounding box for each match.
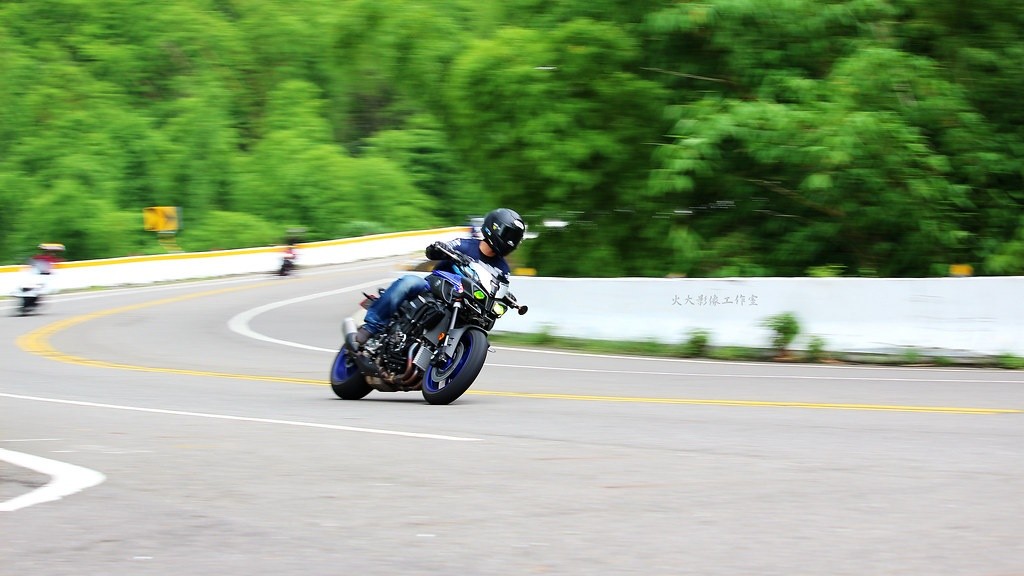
[330,241,528,405]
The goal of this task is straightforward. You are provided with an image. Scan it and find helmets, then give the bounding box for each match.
[481,208,525,257]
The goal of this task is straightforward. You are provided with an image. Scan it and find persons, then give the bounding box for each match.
[351,208,526,352]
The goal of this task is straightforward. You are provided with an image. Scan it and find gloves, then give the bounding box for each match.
[502,293,517,308]
[428,241,447,260]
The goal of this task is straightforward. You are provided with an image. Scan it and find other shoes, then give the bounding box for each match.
[357,328,370,344]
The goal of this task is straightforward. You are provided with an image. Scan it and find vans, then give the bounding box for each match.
[471,218,484,237]
